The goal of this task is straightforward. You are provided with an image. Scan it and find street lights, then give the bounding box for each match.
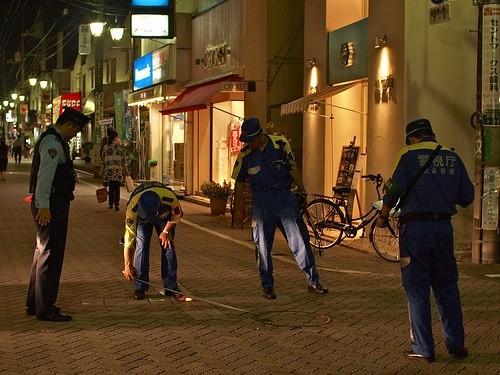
[28,70,53,127]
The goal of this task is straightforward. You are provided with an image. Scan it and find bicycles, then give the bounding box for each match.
[304,173,401,263]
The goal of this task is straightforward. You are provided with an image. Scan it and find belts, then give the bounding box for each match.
[250,186,290,194]
[397,213,453,226]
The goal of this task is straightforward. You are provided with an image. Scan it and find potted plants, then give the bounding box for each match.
[147,160,157,169]
[83,142,94,163]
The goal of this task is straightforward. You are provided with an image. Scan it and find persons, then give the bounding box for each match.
[230,118,328,300]
[376,119,474,360]
[122,183,185,299]
[0,137,9,181]
[10,135,24,164]
[24,106,90,321]
[99,128,131,211]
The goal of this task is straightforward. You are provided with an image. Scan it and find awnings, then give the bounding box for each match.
[279,81,369,120]
[159,75,245,125]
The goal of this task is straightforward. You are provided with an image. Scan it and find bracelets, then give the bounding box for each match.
[163,230,170,235]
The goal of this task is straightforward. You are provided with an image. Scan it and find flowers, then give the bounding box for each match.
[201,180,234,197]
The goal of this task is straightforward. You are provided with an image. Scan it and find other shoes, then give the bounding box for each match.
[165,288,184,296]
[133,289,145,299]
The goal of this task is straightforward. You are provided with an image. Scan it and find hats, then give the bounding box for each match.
[406,119,437,144]
[239,118,262,142]
[61,103,91,134]
[137,191,161,219]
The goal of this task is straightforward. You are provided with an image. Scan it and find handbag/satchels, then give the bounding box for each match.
[95,187,108,204]
[125,176,134,192]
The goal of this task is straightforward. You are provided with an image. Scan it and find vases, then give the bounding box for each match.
[209,195,227,216]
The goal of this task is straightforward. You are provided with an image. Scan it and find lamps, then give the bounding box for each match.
[375,75,395,91]
[374,35,387,49]
[305,57,316,68]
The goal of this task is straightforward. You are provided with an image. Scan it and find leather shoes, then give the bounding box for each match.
[35,312,72,322]
[25,307,62,315]
[307,284,329,295]
[265,290,276,299]
[403,349,435,363]
[447,349,469,357]
